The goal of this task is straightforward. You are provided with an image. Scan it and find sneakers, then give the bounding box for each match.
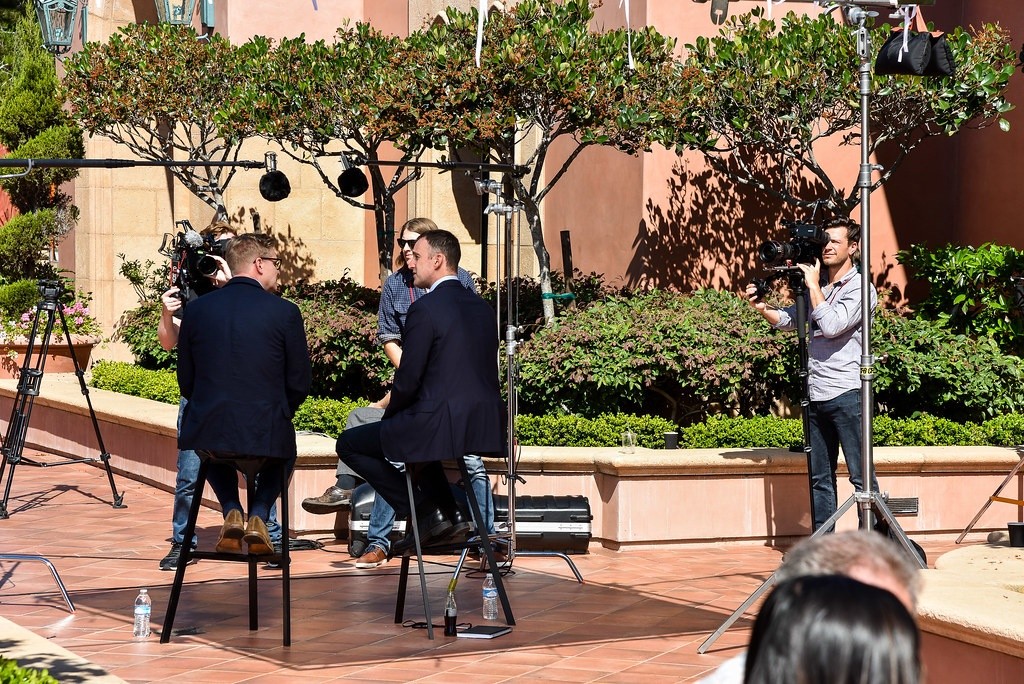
[355,545,388,568]
[159,541,194,571]
[267,544,291,568]
[492,552,512,562]
[302,486,353,515]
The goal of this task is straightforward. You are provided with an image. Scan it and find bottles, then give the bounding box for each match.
[482,574,498,620]
[134,589,151,639]
[444,591,457,637]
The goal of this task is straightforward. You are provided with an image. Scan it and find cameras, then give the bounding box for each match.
[38,280,68,298]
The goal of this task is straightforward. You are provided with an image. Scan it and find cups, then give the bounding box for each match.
[1008,523,1024,548]
[621,433,637,454]
[663,433,678,450]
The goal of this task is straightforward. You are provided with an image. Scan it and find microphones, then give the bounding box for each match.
[184,230,204,247]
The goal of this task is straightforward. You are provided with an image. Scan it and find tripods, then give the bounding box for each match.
[0,297,124,518]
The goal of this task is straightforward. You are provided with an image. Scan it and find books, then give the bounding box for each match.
[456,625,513,639]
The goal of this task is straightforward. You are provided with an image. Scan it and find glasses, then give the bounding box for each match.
[253,257,282,270]
[396,238,417,249]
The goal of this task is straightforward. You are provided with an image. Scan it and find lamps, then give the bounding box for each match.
[34,0,87,64]
[153,0,215,40]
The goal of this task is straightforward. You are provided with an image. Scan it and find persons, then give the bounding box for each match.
[745,215,879,533]
[300,218,509,569]
[689,529,925,684]
[157,220,313,571]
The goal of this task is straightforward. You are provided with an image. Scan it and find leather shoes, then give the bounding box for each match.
[214,509,244,554]
[393,508,453,554]
[244,516,274,555]
[444,507,471,539]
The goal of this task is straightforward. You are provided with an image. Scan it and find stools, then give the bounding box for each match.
[155,450,291,648]
[391,454,514,640]
